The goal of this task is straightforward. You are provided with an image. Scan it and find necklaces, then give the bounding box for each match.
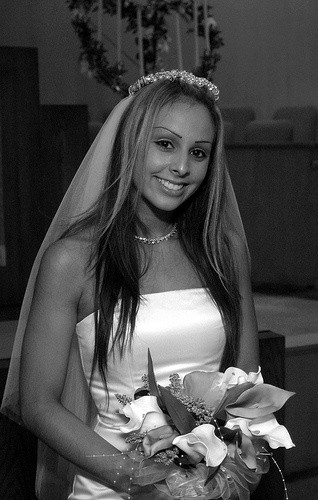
[128,221,179,245]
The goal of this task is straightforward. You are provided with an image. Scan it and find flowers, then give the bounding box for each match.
[65,0,226,97]
[86,348,296,500]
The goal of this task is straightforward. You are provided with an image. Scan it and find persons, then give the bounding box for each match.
[0,69,266,500]
